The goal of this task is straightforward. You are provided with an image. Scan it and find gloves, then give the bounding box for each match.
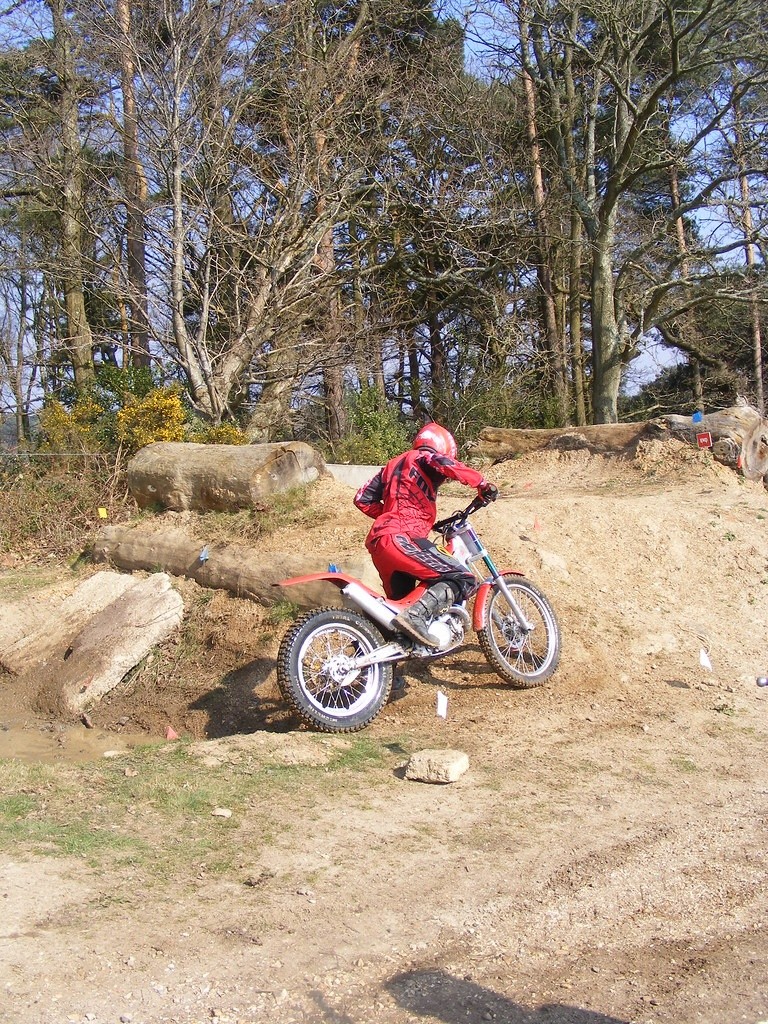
[478,479,500,505]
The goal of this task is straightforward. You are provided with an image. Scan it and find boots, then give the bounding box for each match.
[391,582,455,648]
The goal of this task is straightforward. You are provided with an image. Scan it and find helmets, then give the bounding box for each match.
[412,423,458,460]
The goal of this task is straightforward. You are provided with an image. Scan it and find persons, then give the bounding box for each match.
[353,423,497,648]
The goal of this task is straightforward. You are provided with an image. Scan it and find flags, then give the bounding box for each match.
[437,691,447,719]
[692,411,702,424]
[700,650,712,672]
[200,545,209,561]
[98,508,107,518]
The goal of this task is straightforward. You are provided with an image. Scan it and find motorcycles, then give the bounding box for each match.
[268,483,562,733]
